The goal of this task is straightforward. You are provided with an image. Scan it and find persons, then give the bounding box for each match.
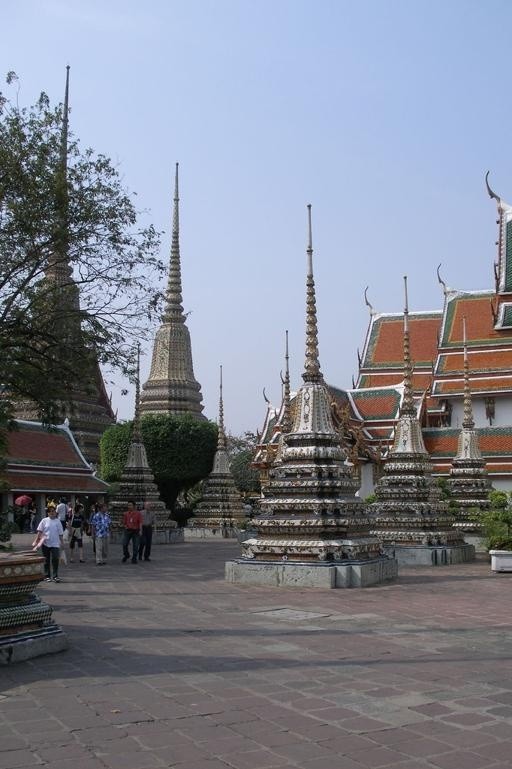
[91,504,112,566]
[19,497,97,534]
[87,508,98,553]
[70,505,86,564]
[244,502,252,517]
[138,501,154,561]
[33,507,65,582]
[121,500,144,564]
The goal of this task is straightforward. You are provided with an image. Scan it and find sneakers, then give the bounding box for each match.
[69,557,153,568]
[52,577,63,583]
[44,578,52,582]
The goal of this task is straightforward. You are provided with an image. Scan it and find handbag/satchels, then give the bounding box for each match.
[65,514,69,523]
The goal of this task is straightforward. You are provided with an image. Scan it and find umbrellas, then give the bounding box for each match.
[15,495,32,506]
